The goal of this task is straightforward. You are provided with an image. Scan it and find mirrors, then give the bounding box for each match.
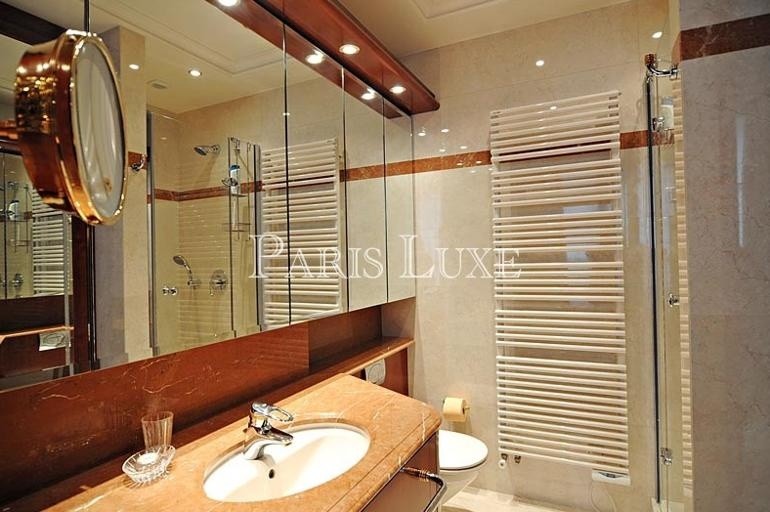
[0,0,417,394]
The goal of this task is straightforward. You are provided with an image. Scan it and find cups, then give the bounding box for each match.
[141,410,173,449]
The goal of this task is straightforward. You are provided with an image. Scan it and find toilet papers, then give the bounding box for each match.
[441,396,468,423]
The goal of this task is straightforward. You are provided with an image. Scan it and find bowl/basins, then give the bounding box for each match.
[121,444,175,483]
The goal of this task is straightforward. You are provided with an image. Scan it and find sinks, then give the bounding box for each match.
[203,417,370,504]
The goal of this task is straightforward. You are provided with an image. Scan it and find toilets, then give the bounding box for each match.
[437,429,490,505]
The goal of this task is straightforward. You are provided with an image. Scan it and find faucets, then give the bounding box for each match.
[244,401,293,461]
